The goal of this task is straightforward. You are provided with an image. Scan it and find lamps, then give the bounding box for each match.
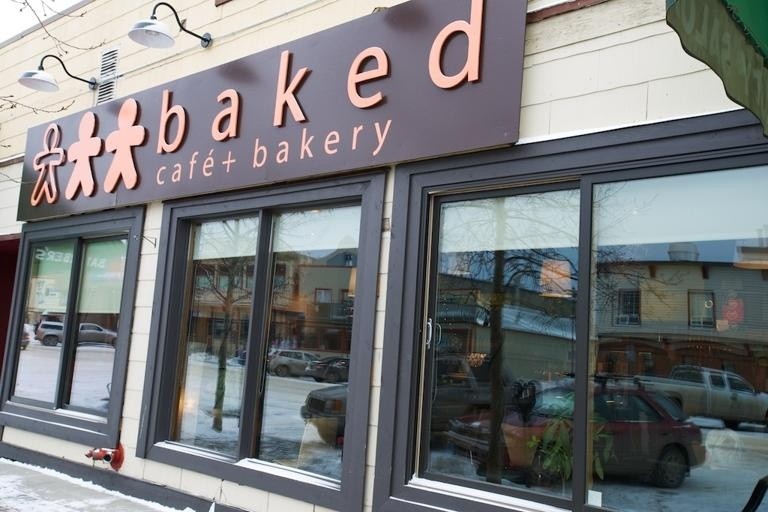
[538,255,572,296]
[128,2,211,48]
[18,55,98,92]
[346,268,357,295]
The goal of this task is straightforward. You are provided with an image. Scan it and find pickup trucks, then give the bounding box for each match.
[302,352,521,453]
[606,362,767,430]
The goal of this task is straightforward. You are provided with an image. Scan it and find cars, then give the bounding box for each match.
[447,374,705,490]
[21,320,118,350]
[238,346,350,384]
